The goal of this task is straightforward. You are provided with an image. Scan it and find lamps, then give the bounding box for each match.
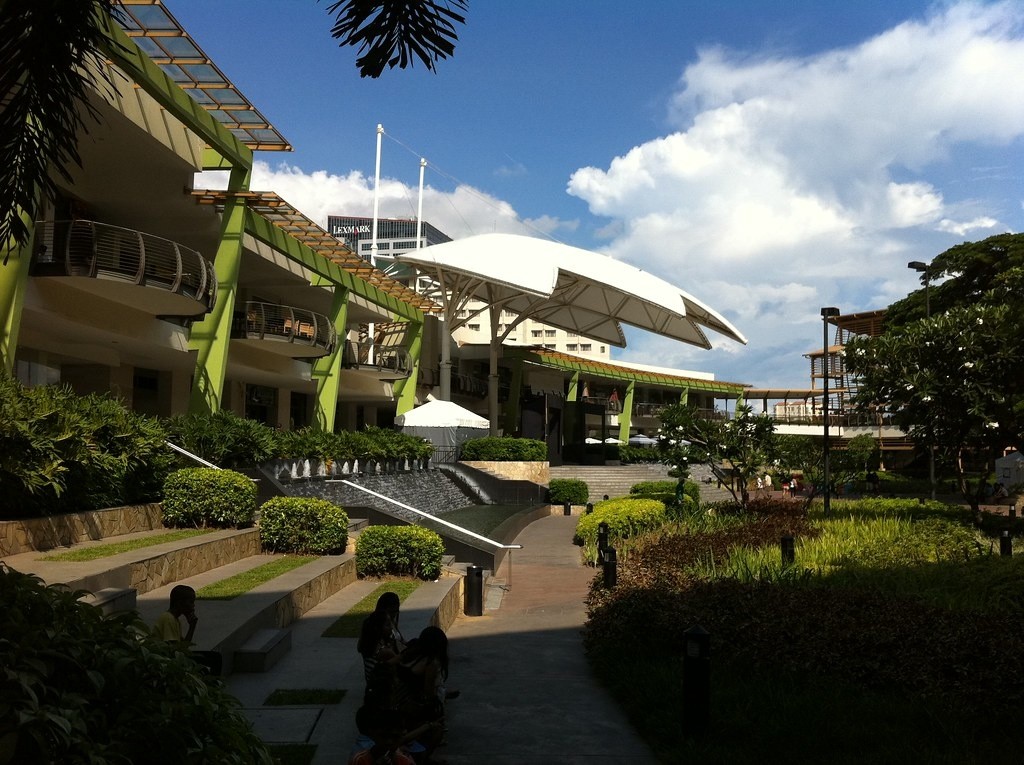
[1000,527,1012,557]
[1009,504,1016,524]
[603,546,616,587]
[598,522,609,564]
[586,503,593,515]
[781,534,794,566]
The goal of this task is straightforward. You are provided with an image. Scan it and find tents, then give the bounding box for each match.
[400,401,490,462]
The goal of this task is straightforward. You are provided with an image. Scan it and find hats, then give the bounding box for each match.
[403,738,426,753]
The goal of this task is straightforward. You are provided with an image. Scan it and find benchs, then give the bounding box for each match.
[984,497,1018,506]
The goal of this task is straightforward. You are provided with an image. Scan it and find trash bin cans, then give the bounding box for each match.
[466,566,484,616]
[564,496,570,515]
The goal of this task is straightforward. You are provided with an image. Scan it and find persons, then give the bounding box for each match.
[779,470,798,499]
[676,477,685,499]
[148,586,223,682]
[753,472,773,501]
[850,471,879,495]
[356,593,462,765]
[982,482,1008,505]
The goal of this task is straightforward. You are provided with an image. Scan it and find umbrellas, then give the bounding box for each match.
[630,434,691,445]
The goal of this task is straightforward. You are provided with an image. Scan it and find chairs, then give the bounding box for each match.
[814,412,880,426]
[240,312,318,339]
